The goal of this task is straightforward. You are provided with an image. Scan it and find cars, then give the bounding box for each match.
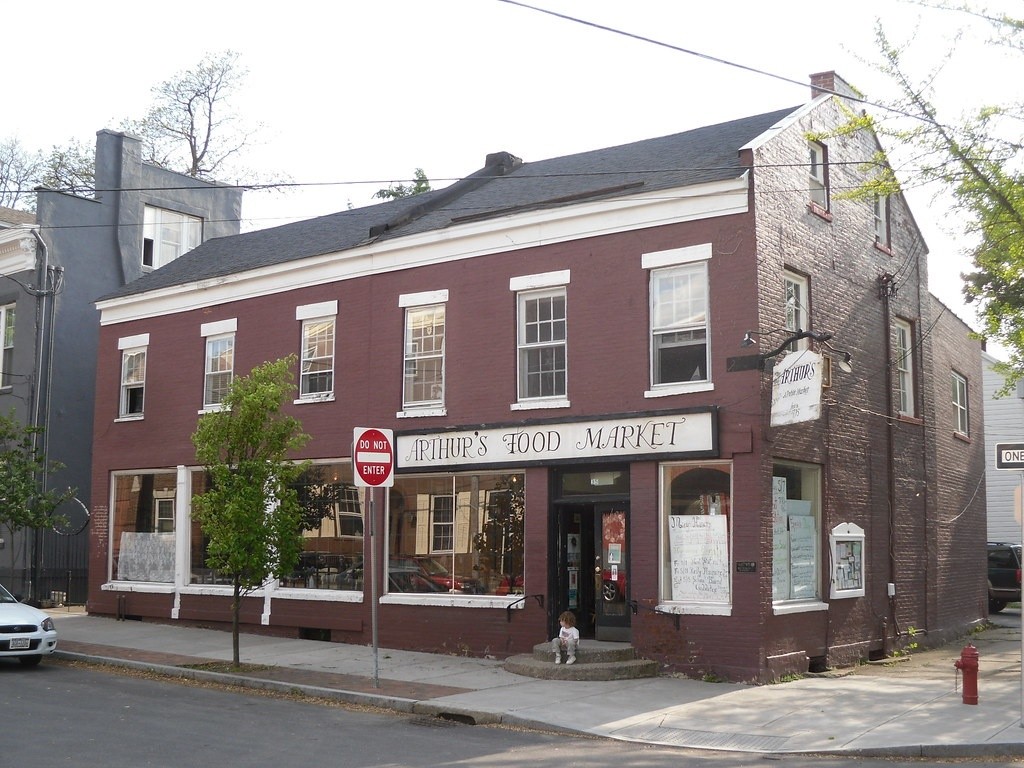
[0,584,58,666]
[602,568,625,604]
[285,553,524,596]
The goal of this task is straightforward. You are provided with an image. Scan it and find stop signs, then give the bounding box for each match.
[353,427,394,487]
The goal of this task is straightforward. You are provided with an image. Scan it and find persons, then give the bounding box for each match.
[472,557,490,593]
[348,561,358,591]
[336,555,347,590]
[551,610,579,665]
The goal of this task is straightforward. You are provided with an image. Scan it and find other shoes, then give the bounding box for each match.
[555,657,562,664]
[566,656,576,664]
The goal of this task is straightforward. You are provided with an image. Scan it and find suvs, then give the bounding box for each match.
[987,541,1023,611]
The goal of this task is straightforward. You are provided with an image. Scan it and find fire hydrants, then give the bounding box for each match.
[954,642,979,705]
